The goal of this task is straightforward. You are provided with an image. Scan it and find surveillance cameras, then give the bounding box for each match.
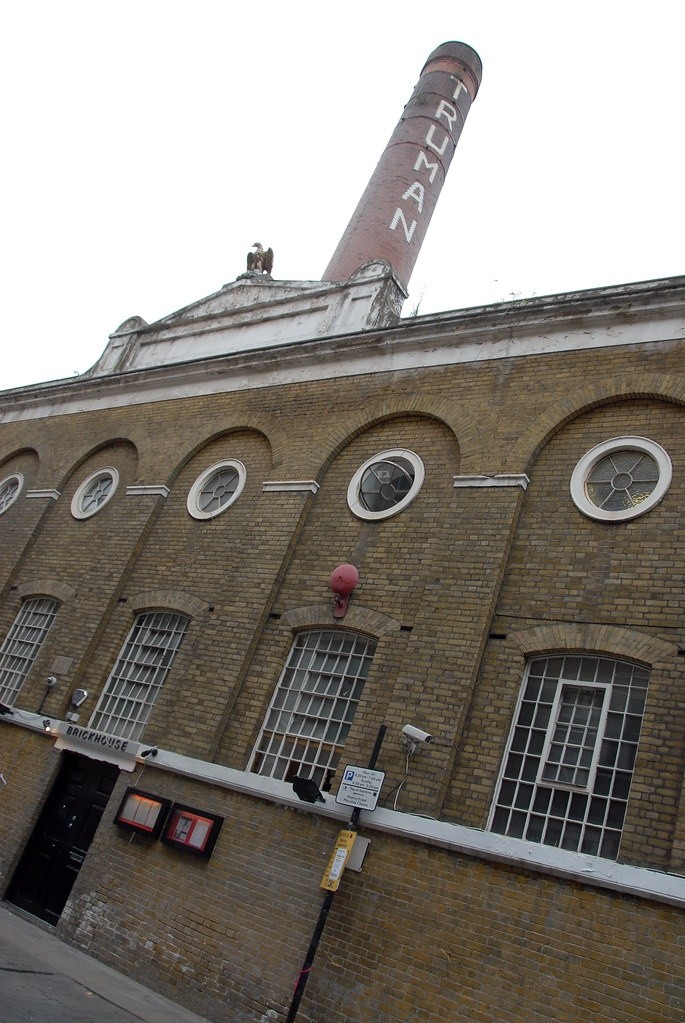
[46,676,56,687]
[402,724,433,746]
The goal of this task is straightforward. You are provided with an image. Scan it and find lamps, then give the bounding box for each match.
[292,775,326,804]
[0,703,13,715]
[141,749,158,757]
[47,677,56,686]
[43,720,50,732]
